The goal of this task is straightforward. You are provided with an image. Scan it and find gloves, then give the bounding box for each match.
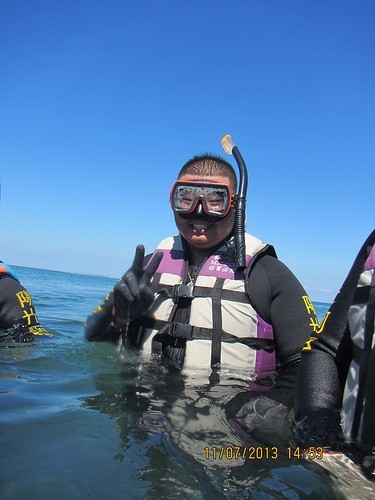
[113,244,165,320]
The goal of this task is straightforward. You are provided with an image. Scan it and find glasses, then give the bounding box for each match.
[168,180,235,220]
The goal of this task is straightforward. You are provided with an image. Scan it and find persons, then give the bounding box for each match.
[1,261,45,333]
[84,154,320,434]
[293,228,374,458]
